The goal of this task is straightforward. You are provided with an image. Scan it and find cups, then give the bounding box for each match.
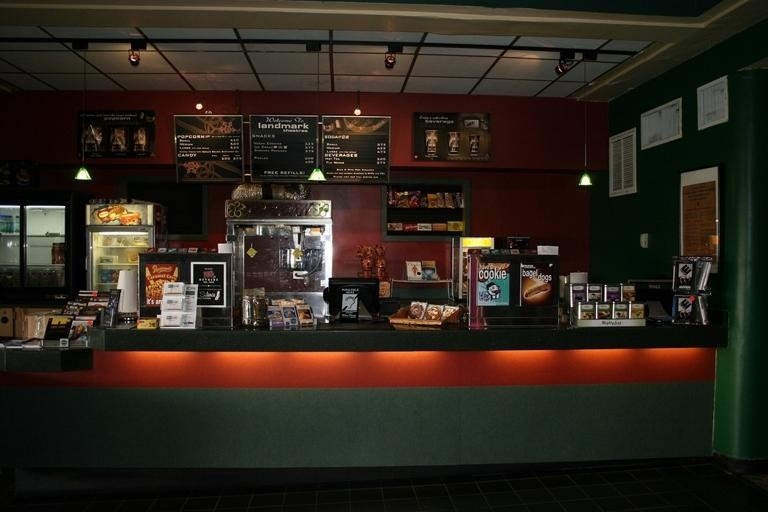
[426,130,480,157]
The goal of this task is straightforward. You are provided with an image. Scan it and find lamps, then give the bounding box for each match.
[127,37,576,76]
[306,52,328,181]
[576,59,600,188]
[73,51,93,182]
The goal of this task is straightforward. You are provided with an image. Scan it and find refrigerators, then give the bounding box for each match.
[86,203,167,296]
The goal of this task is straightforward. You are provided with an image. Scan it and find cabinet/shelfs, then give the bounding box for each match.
[473,251,561,332]
[0,190,86,343]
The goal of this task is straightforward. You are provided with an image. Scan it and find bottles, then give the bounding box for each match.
[51,243,65,264]
[0,215,20,233]
[241,296,268,324]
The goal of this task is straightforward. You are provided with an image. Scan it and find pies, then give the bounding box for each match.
[523,277,551,300]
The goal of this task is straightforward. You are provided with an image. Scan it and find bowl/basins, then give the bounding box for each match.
[168,234,182,240]
